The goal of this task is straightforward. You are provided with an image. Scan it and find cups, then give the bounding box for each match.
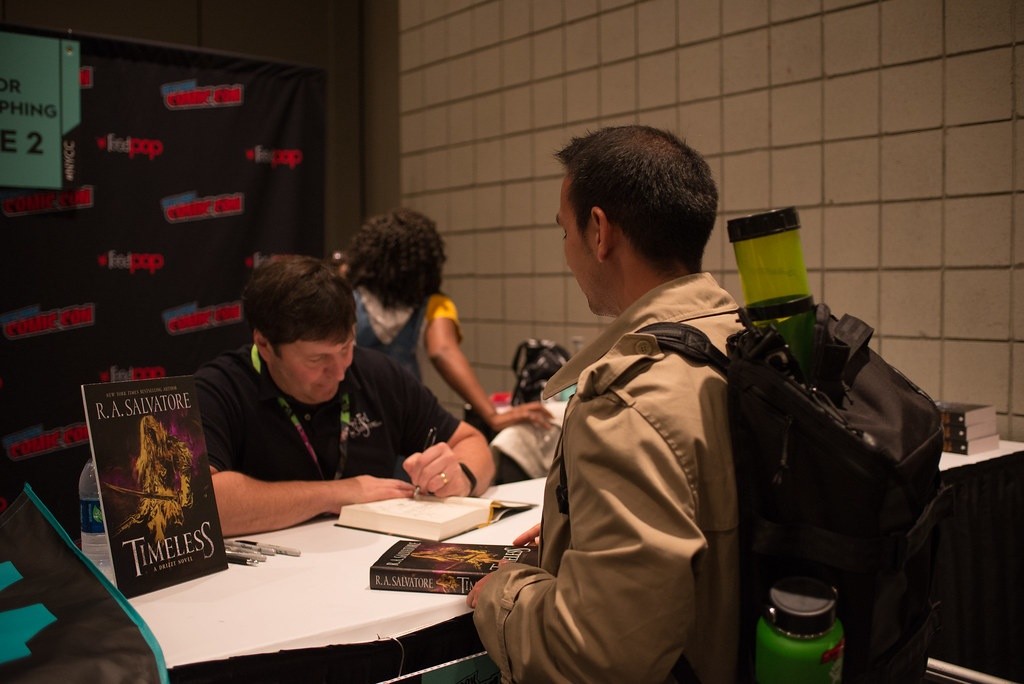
[729,206,813,384]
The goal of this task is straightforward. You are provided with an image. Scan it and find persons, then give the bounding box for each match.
[466,122,757,684]
[332,206,552,435]
[190,254,497,538]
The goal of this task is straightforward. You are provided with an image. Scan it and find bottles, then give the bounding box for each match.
[561,336,585,400]
[755,574,844,684]
[78,454,116,581]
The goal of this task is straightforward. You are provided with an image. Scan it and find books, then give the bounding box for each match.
[333,484,541,543]
[928,399,1002,458]
[369,539,547,595]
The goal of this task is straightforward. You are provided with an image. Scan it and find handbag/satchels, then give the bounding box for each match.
[510,338,574,409]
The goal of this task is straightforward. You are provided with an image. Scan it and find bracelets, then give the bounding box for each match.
[459,462,477,497]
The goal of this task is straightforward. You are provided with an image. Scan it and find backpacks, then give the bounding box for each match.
[637,301,954,684]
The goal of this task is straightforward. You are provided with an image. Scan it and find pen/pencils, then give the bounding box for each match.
[224,539,302,565]
[414,426,438,496]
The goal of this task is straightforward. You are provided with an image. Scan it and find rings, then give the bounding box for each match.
[439,472,447,484]
